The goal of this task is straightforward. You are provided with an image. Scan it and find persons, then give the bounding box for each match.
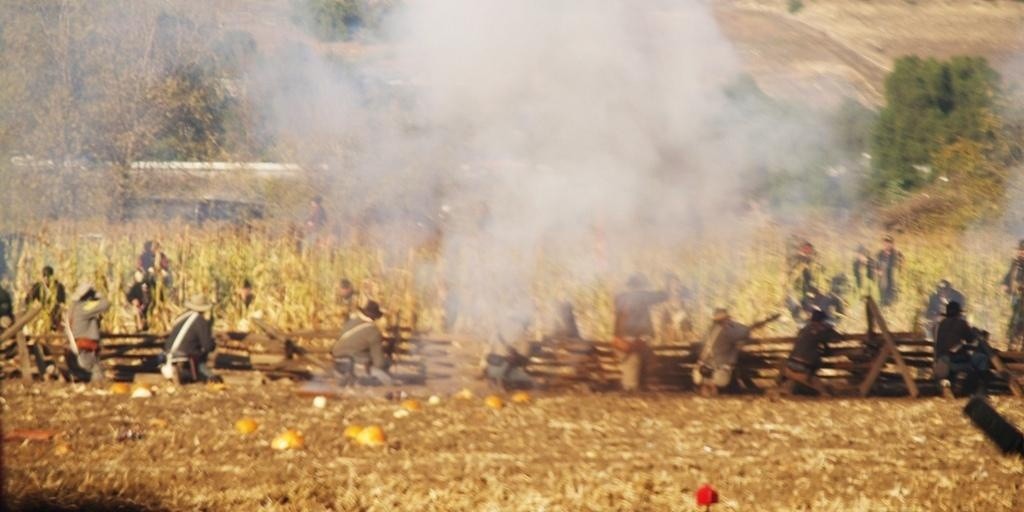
[329,301,396,388]
[549,289,583,340]
[137,240,172,289]
[768,309,848,402]
[25,266,65,329]
[479,309,533,393]
[691,306,750,397]
[236,280,254,307]
[612,275,668,391]
[999,240,1023,352]
[335,278,359,306]
[304,195,327,231]
[161,294,225,386]
[782,232,906,328]
[656,273,697,347]
[426,282,457,334]
[933,301,990,402]
[126,265,153,333]
[46,284,111,382]
[918,280,966,339]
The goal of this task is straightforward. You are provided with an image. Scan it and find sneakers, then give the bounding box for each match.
[941,382,955,402]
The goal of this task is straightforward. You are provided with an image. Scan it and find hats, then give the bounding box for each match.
[68,279,98,302]
[804,309,835,324]
[182,294,212,313]
[711,307,732,322]
[356,298,384,320]
[941,300,966,317]
